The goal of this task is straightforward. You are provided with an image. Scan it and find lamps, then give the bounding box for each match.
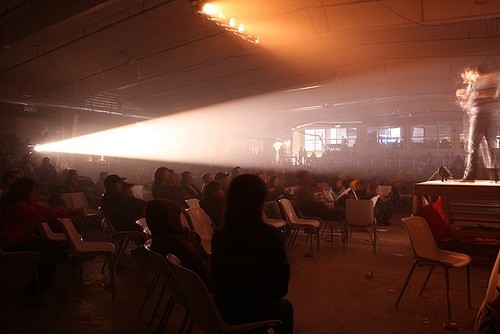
[438,165,452,181]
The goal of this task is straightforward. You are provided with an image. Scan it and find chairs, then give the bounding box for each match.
[0,183,411,334]
[413,199,456,249]
[396,216,473,319]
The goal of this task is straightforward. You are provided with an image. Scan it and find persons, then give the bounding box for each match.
[103,175,150,246]
[456,65,499,183]
[145,199,216,295]
[152,136,466,222]
[211,174,294,334]
[0,157,150,307]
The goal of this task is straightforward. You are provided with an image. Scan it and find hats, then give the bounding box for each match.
[215,172,231,180]
[121,182,135,188]
[104,175,127,186]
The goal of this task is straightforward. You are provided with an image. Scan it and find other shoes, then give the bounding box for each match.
[23,294,41,306]
